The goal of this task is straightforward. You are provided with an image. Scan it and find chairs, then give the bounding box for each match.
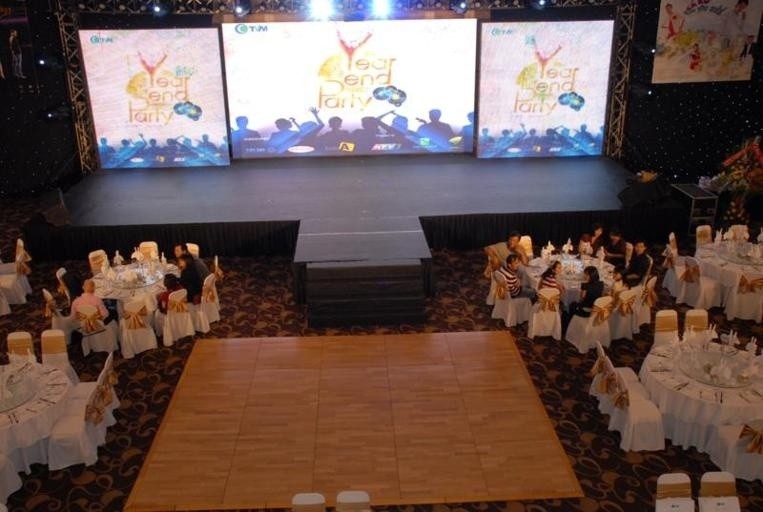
[655,473,695,512]
[336,491,371,512]
[699,471,740,512]
[292,493,327,511]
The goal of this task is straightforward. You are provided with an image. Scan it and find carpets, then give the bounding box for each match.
[122,331,584,511]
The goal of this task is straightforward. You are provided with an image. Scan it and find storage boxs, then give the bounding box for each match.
[670,183,719,232]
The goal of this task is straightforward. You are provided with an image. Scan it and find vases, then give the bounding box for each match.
[723,192,751,229]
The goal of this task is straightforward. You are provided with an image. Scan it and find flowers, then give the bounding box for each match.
[721,134,763,198]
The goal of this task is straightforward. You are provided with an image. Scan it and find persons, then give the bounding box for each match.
[664,0,757,73]
[738,168,763,243]
[485,223,650,335]
[59,243,210,332]
[9,29,27,79]
